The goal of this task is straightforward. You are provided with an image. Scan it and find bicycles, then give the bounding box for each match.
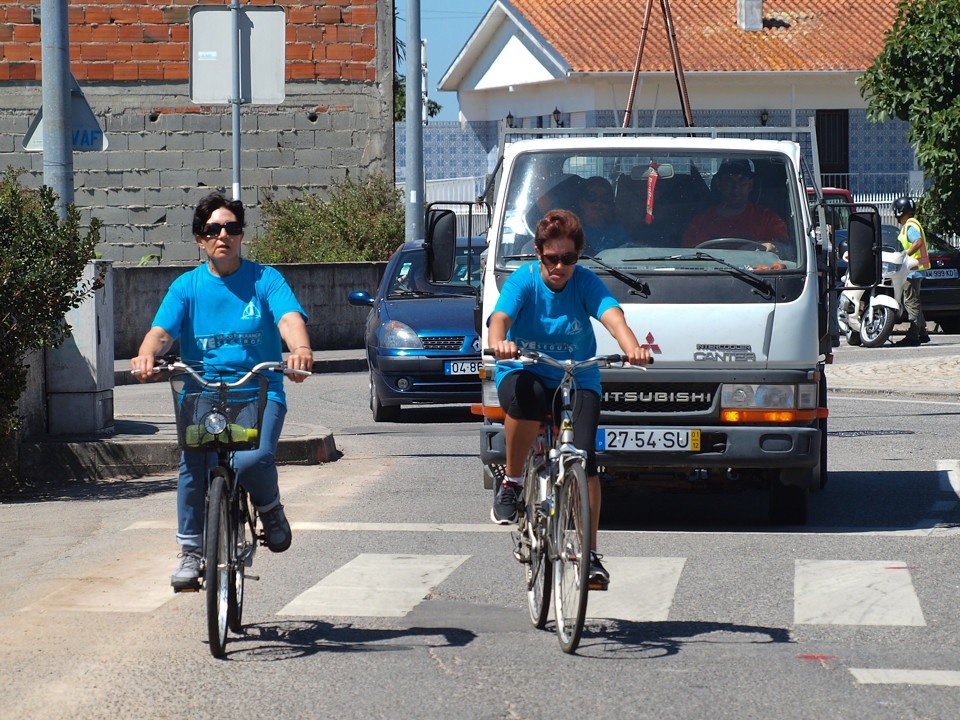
[482,346,655,654]
[132,354,312,658]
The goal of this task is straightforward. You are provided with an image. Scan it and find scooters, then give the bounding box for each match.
[837,239,919,348]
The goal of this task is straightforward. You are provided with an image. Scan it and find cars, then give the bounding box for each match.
[347,234,489,422]
[804,187,959,335]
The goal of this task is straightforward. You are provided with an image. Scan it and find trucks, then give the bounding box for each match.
[422,113,884,530]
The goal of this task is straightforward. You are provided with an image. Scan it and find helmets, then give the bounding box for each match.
[892,197,916,218]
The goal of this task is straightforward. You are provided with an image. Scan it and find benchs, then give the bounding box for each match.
[537,174,764,248]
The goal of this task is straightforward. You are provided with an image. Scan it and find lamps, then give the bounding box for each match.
[762,109,770,126]
[553,107,566,128]
[506,110,518,128]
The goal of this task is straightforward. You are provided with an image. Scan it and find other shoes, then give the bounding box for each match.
[897,338,921,347]
[919,335,931,343]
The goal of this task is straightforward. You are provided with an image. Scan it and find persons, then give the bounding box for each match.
[888,196,932,347]
[485,209,651,584]
[533,155,633,258]
[678,161,791,259]
[130,192,313,587]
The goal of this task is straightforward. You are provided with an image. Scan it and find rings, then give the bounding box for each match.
[301,363,307,366]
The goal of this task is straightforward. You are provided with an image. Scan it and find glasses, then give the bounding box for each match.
[540,252,578,266]
[584,190,612,204]
[199,221,243,238]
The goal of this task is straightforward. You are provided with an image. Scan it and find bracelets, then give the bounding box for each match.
[290,345,311,351]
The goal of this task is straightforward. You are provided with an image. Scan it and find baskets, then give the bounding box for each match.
[168,371,269,455]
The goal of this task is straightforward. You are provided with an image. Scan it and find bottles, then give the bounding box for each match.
[186,424,258,447]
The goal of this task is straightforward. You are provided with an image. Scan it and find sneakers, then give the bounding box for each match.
[490,479,523,525]
[257,501,292,553]
[170,545,201,589]
[577,552,610,584]
